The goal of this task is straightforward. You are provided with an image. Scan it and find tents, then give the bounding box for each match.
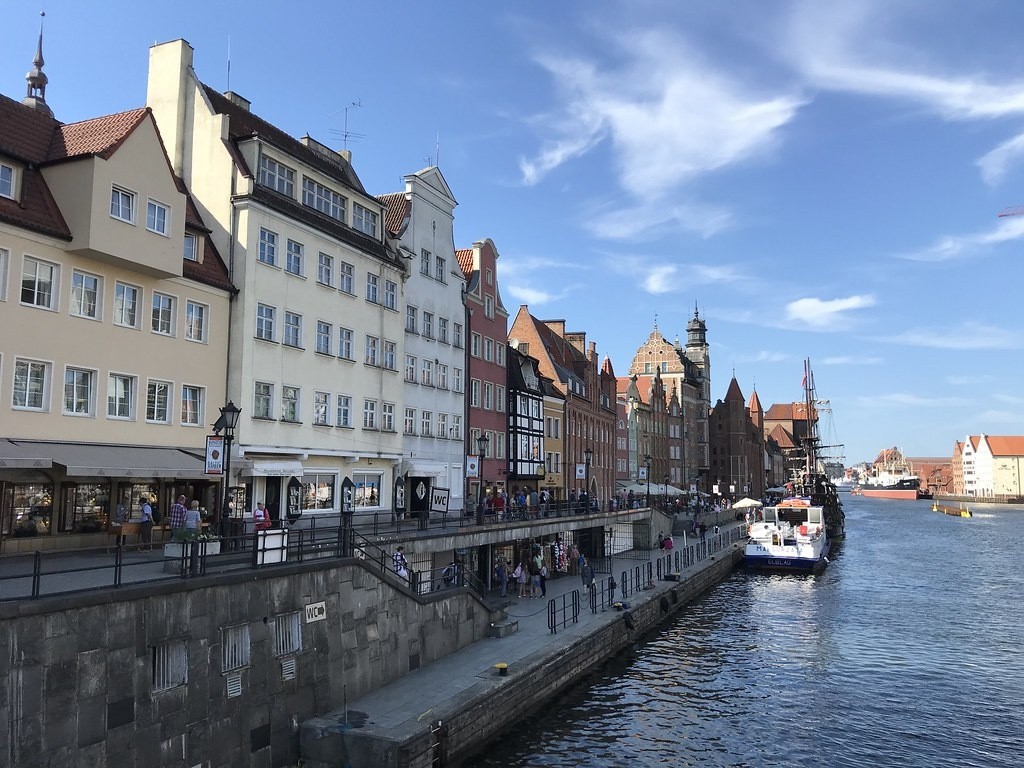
[732,497,764,513]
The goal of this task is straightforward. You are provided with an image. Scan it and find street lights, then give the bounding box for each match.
[663,474,670,513]
[217,400,243,537]
[645,455,653,508]
[473,431,490,526]
[747,480,752,498]
[694,476,700,513]
[583,447,594,515]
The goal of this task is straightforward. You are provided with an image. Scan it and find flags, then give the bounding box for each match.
[802,373,806,388]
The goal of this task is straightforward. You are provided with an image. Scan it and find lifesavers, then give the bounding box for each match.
[814,493,826,504]
[672,590,678,603]
[625,611,634,629]
[662,596,668,612]
[442,567,453,582]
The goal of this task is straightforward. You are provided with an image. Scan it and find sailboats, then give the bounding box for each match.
[769,354,847,545]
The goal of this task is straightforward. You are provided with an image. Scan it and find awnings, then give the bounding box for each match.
[241,454,303,477]
[402,460,446,476]
[0,438,255,478]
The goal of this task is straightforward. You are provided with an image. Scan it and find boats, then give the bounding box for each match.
[739,469,834,579]
[851,472,935,501]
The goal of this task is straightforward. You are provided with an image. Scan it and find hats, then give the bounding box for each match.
[257,500,264,503]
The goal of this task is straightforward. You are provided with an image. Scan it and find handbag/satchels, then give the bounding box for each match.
[545,574,550,579]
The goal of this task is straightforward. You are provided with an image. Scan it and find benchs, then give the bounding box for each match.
[489,618,520,638]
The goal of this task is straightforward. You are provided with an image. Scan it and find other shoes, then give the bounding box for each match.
[501,595,507,598]
[138,545,150,550]
[518,596,520,598]
[540,594,545,598]
[529,596,532,599]
[583,593,586,595]
[534,596,537,599]
[524,595,526,597]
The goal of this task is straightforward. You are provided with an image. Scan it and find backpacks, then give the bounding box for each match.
[495,565,504,577]
[142,503,161,522]
[444,567,453,583]
[715,527,718,533]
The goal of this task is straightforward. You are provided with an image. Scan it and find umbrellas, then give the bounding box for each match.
[765,487,785,497]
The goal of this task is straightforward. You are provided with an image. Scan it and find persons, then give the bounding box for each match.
[659,531,673,551]
[610,489,646,512]
[254,502,269,529]
[499,552,546,599]
[464,491,528,524]
[758,496,770,505]
[530,489,553,518]
[735,510,757,524]
[570,546,595,595]
[442,563,455,586]
[675,497,727,516]
[116,495,202,553]
[694,522,720,544]
[570,489,597,512]
[392,546,408,577]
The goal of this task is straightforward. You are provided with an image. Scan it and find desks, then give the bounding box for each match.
[151,522,211,549]
[107,522,154,555]
